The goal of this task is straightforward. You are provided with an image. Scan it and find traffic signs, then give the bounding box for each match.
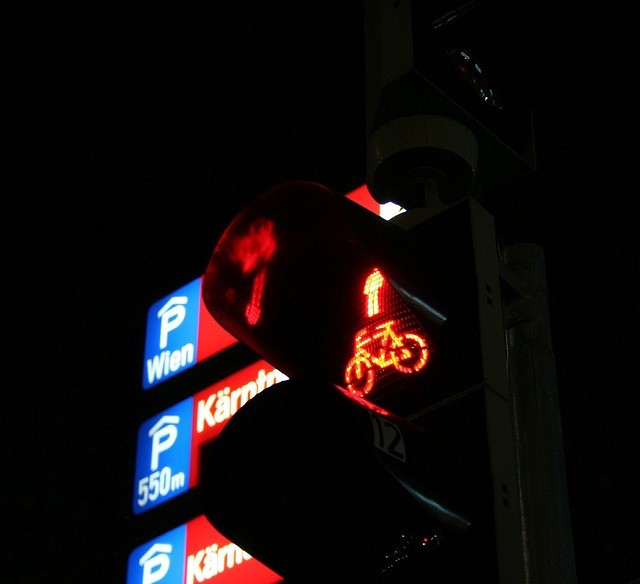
[126,515,285,584]
[133,358,289,515]
[143,276,244,391]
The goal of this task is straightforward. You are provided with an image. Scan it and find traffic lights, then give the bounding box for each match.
[196,197,502,584]
